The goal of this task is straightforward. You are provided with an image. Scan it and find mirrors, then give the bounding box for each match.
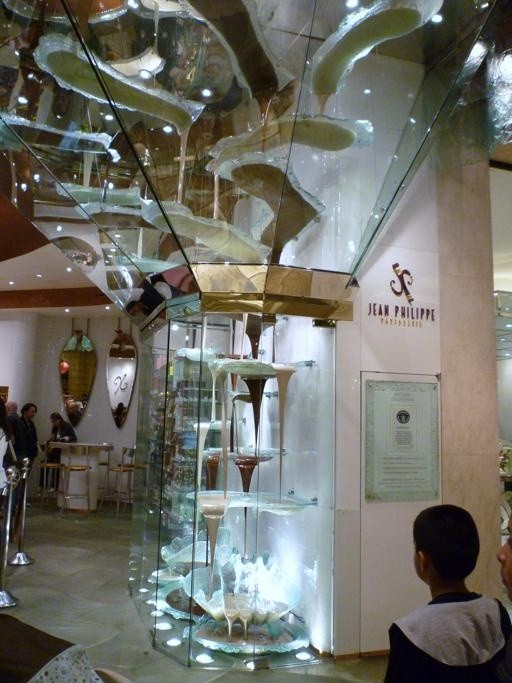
[59,327,138,430]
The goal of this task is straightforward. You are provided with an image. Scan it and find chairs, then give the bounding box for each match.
[33,440,137,520]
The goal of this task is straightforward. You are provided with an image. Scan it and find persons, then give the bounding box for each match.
[497,511,512,602]
[37,412,77,492]
[384,505,512,683]
[0,398,37,542]
